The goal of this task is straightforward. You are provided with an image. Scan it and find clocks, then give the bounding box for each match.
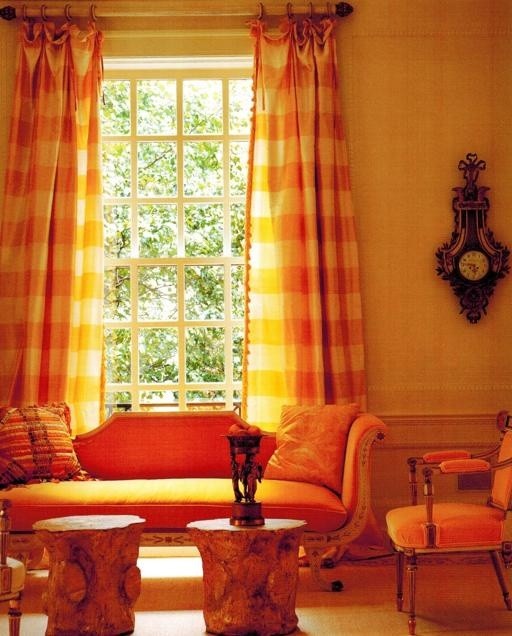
[434,152,512,325]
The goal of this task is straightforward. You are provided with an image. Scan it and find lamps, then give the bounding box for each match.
[217,417,269,528]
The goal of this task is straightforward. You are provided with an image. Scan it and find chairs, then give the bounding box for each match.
[384,410,511,636]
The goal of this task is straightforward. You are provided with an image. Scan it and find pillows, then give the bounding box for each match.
[263,399,361,495]
[1,399,102,491]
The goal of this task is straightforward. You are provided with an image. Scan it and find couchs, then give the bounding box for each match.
[0,400,383,594]
